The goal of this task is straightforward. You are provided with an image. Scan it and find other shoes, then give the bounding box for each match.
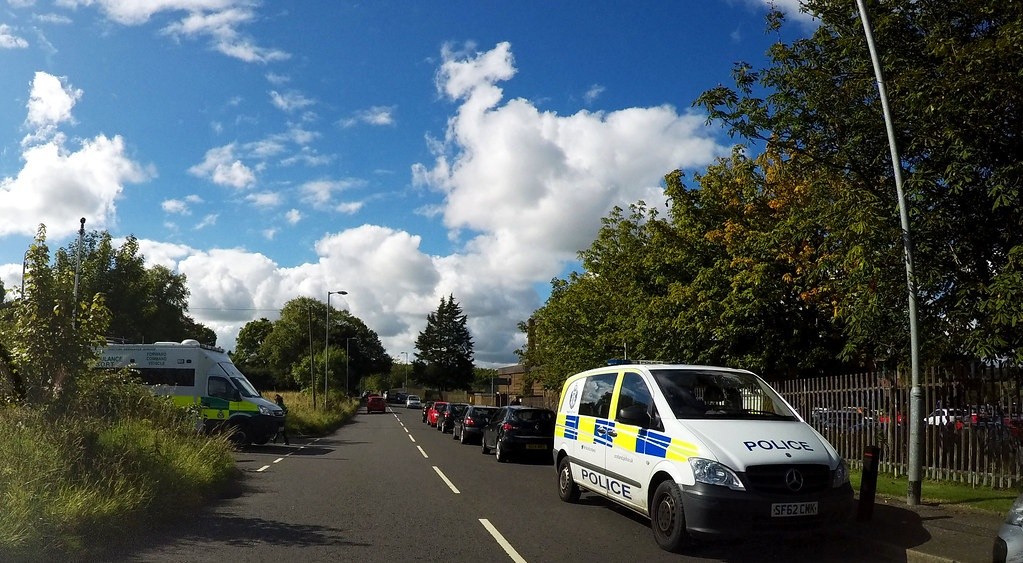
[283,443,290,446]
[269,438,276,444]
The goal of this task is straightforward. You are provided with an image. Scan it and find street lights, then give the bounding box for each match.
[345,337,358,399]
[401,351,408,393]
[324,291,348,409]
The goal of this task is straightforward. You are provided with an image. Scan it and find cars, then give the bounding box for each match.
[481,405,556,463]
[813,405,909,429]
[437,403,469,433]
[453,405,501,445]
[426,402,451,427]
[923,408,965,429]
[964,412,997,429]
[405,395,423,409]
[422,401,435,423]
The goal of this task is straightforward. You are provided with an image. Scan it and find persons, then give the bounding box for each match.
[270,393,290,445]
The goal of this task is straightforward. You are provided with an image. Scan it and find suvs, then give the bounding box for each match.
[366,393,380,404]
[395,392,408,404]
[367,397,387,414]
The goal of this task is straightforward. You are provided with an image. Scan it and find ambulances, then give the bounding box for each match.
[80,339,285,446]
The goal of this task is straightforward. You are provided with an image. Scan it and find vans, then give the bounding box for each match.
[553,359,851,553]
